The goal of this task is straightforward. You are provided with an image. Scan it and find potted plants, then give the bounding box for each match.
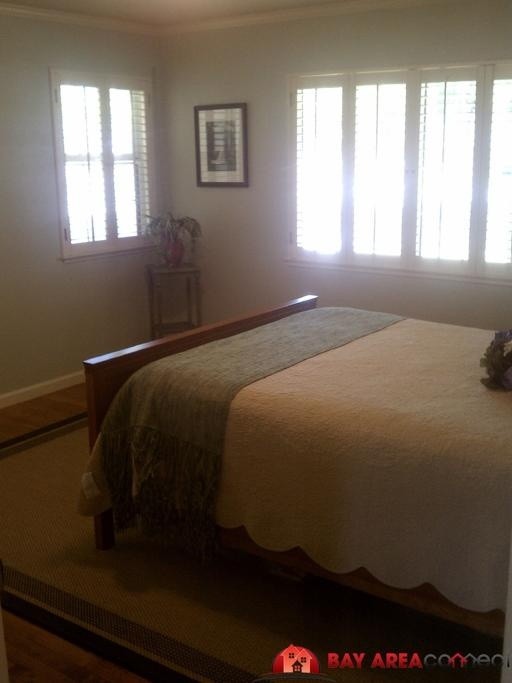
[142,210,202,266]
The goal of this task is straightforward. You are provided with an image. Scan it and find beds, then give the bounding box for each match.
[81,293,512,554]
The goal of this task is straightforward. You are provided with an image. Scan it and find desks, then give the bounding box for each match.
[144,268,201,342]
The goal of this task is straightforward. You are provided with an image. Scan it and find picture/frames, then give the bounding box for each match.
[194,101,248,188]
